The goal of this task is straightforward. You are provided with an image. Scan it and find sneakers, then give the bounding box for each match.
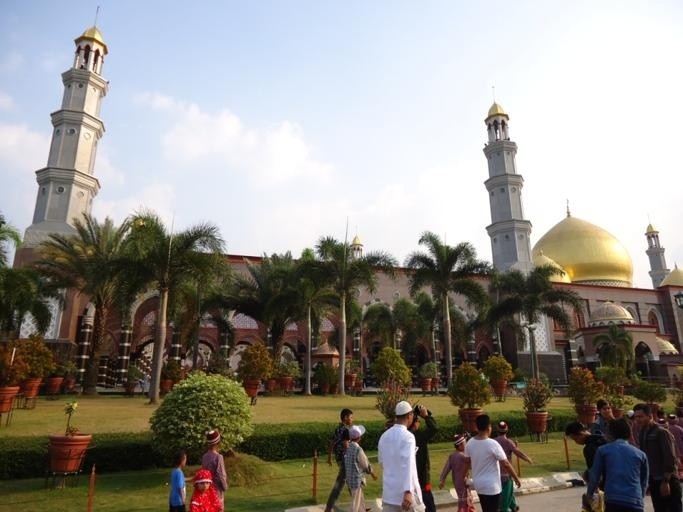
[366,507,372,511]
[512,507,519,512]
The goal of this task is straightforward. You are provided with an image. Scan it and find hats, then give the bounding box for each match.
[668,413,678,421]
[206,431,221,445]
[192,470,213,483]
[626,411,634,421]
[348,425,365,439]
[453,434,466,445]
[396,400,413,415]
[497,422,508,432]
[658,419,668,427]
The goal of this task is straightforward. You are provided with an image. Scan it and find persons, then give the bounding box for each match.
[344,424,377,512]
[493,421,534,512]
[324,409,353,512]
[377,400,426,511]
[168,449,187,512]
[408,403,436,512]
[190,469,223,512]
[463,414,521,512]
[439,434,478,512]
[564,399,683,512]
[202,430,227,501]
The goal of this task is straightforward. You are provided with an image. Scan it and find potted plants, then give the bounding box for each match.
[567,366,683,425]
[160,359,180,392]
[48,401,92,474]
[483,352,513,396]
[521,378,553,433]
[237,341,441,397]
[447,361,493,433]
[0,334,74,413]
